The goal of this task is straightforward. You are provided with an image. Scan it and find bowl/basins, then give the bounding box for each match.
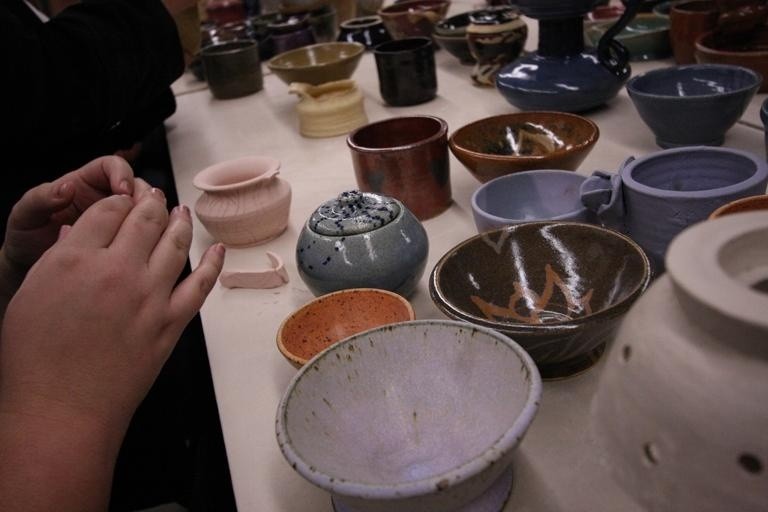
[277,288,418,368]
[445,108,601,182]
[275,320,543,512]
[192,154,292,247]
[190,2,764,149]
[429,220,652,382]
[471,169,616,233]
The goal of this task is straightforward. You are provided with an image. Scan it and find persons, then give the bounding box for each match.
[1,152,227,512]
[0,0,185,236]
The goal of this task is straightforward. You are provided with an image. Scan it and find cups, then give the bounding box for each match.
[344,115,454,222]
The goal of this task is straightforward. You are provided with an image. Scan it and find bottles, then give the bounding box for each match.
[621,146,767,264]
[567,210,768,512]
[296,190,429,298]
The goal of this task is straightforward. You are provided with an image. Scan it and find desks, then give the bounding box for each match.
[155,16,767,512]
